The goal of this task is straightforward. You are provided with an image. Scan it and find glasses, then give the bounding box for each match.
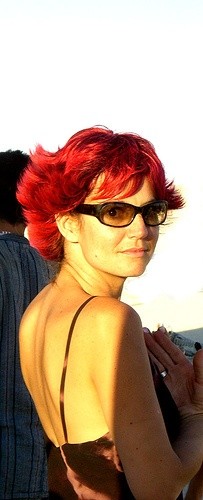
[74,199,169,228]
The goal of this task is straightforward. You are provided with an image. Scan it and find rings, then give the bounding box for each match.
[160,371,168,377]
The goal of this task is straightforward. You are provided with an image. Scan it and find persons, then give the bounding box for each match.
[14,126,203,500]
[0,148,53,500]
